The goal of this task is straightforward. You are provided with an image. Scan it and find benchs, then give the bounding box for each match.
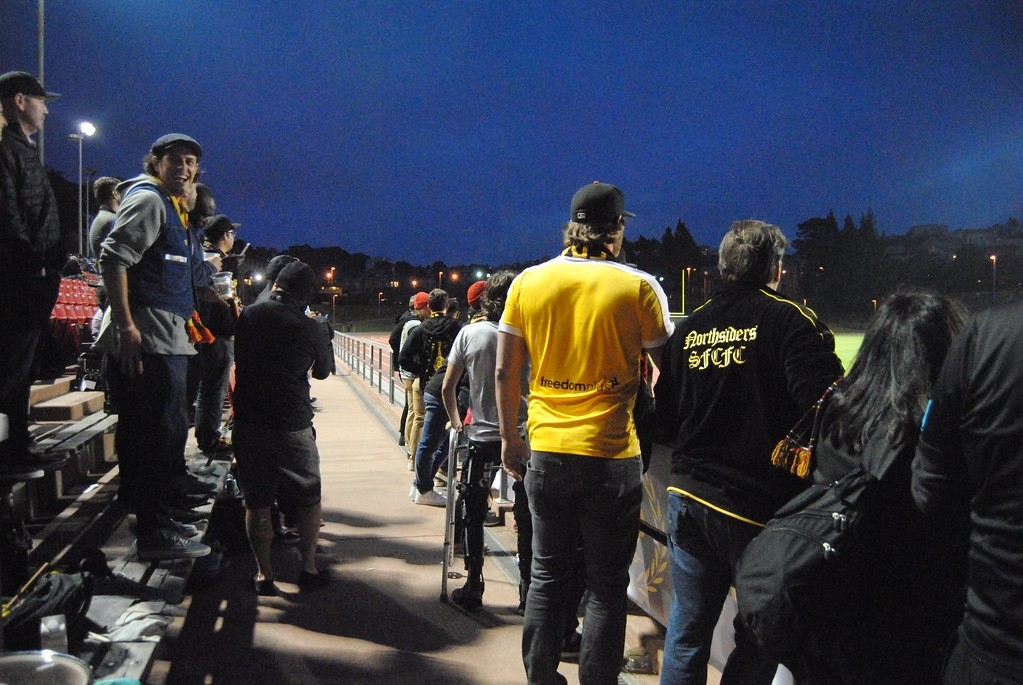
[0,407,234,685]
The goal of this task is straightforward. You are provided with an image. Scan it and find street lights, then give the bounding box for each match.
[990,255,996,301]
[378,292,383,320]
[67,122,96,254]
[86,168,98,259]
[332,294,339,328]
[439,272,444,289]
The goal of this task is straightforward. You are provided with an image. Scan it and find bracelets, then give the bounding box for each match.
[120,326,134,332]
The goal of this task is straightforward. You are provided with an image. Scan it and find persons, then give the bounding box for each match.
[772,290,972,478]
[654,219,844,685]
[64,254,86,280]
[494,180,675,685]
[442,271,532,614]
[911,302,1022,684]
[409,366,470,505]
[90,133,243,556]
[231,255,336,595]
[0,71,71,478]
[389,289,465,472]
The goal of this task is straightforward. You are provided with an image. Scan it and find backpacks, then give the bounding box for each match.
[735,403,918,685]
[419,332,455,394]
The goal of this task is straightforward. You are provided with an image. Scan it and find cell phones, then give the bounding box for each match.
[241,243,250,255]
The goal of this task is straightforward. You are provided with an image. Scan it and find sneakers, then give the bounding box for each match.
[558,632,582,658]
[269,530,299,544]
[410,484,448,506]
[484,513,503,526]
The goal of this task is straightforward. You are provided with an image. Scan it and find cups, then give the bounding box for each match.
[1,652,92,685]
[210,271,234,301]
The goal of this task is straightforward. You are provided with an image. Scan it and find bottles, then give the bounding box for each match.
[105,392,112,412]
[225,476,237,498]
[73,368,104,391]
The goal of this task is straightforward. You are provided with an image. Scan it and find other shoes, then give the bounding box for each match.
[297,566,330,591]
[141,475,216,559]
[453,583,484,605]
[205,438,234,454]
[248,571,275,596]
[20,453,71,473]
[436,469,451,484]
[0,460,45,480]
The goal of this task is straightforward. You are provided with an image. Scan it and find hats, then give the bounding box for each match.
[266,255,295,280]
[276,262,316,296]
[468,281,487,306]
[0,71,61,103]
[153,133,202,157]
[204,215,240,238]
[414,292,429,305]
[571,184,637,223]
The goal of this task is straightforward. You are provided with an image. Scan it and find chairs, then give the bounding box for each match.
[51,278,103,350]
